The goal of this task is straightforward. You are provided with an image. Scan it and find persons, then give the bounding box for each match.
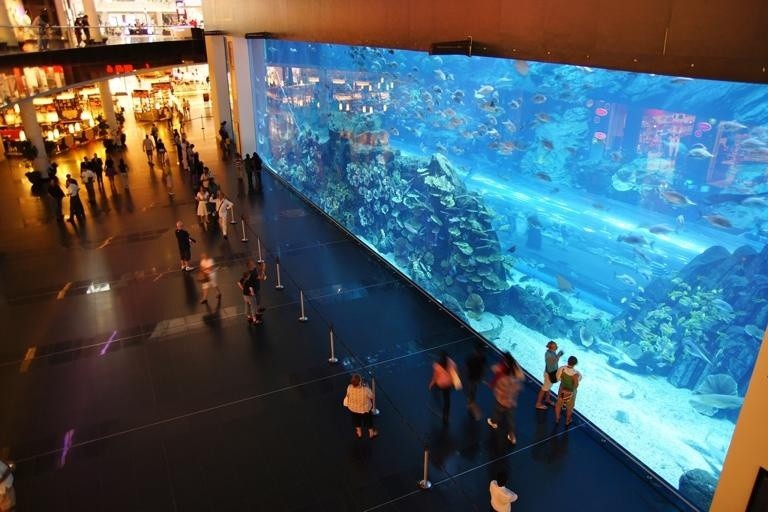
[460,339,490,423]
[0,447,20,512]
[487,366,517,446]
[38,6,91,52]
[488,465,519,512]
[143,120,270,329]
[535,340,564,411]
[488,351,525,388]
[553,354,583,426]
[341,373,380,439]
[428,348,465,427]
[43,107,130,232]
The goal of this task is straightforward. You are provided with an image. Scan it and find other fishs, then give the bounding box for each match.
[264,34,768,417]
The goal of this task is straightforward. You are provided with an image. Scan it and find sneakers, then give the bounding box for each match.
[507,434,516,443]
[216,293,220,297]
[200,298,208,303]
[181,265,194,270]
[487,418,497,428]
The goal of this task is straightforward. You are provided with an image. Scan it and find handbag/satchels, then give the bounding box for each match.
[211,210,219,219]
[450,367,462,390]
[549,369,558,383]
[343,396,350,407]
